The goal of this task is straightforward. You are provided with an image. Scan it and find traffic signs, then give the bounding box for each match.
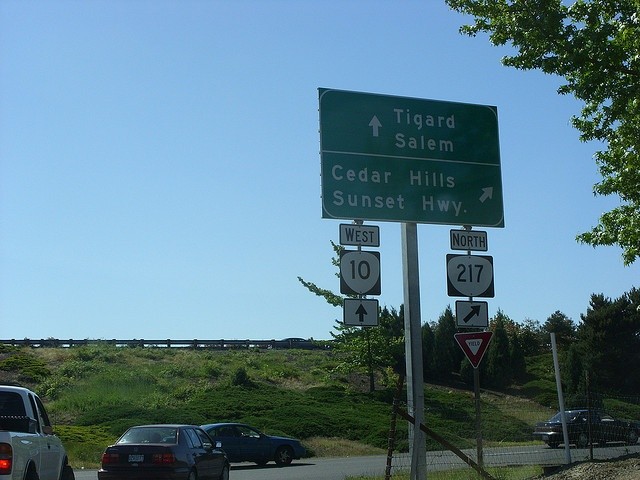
[448,228,488,252]
[318,151,505,229]
[315,87,502,166]
[338,223,379,247]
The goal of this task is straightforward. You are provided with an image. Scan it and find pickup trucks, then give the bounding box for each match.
[0,381,77,480]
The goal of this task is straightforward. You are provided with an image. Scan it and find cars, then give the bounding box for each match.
[97,423,231,480]
[533,408,640,449]
[199,423,306,467]
[271,337,317,350]
